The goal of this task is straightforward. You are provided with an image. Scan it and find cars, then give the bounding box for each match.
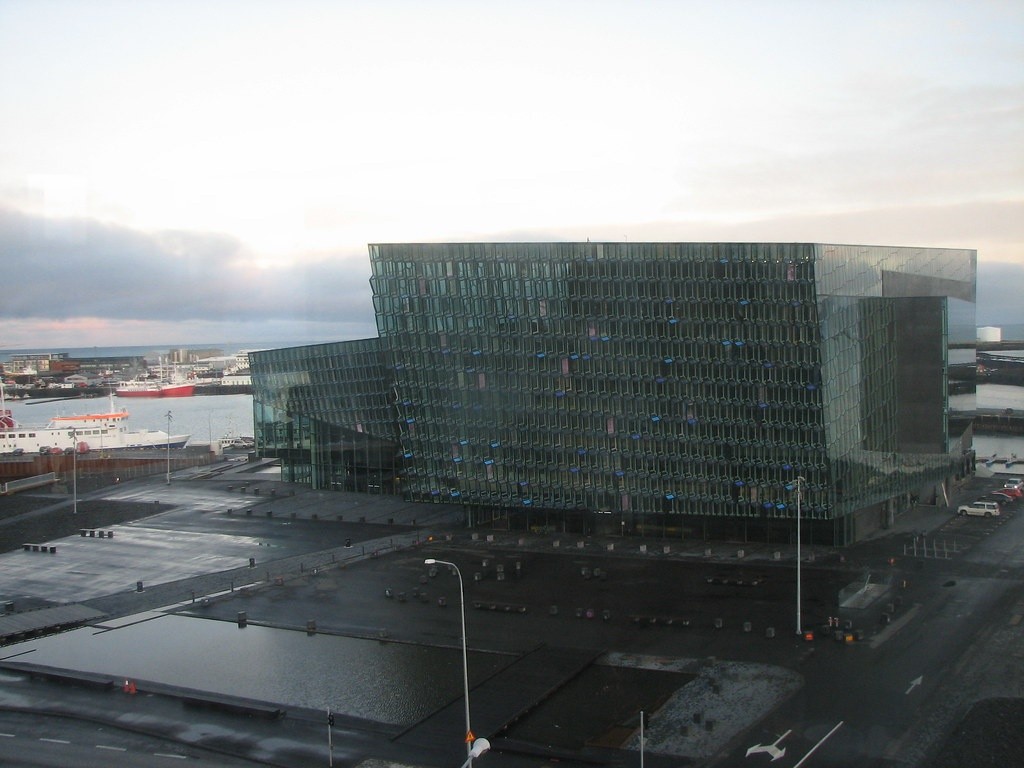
[967,492,1013,507]
[991,488,1022,497]
[1003,478,1024,489]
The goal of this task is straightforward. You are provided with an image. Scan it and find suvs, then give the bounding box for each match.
[957,501,1000,519]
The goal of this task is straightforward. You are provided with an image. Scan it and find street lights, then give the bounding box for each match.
[67,431,82,515]
[792,475,811,638]
[166,410,175,486]
[424,557,479,768]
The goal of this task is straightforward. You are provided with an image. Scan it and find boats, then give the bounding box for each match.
[115,370,195,398]
[0,391,191,454]
